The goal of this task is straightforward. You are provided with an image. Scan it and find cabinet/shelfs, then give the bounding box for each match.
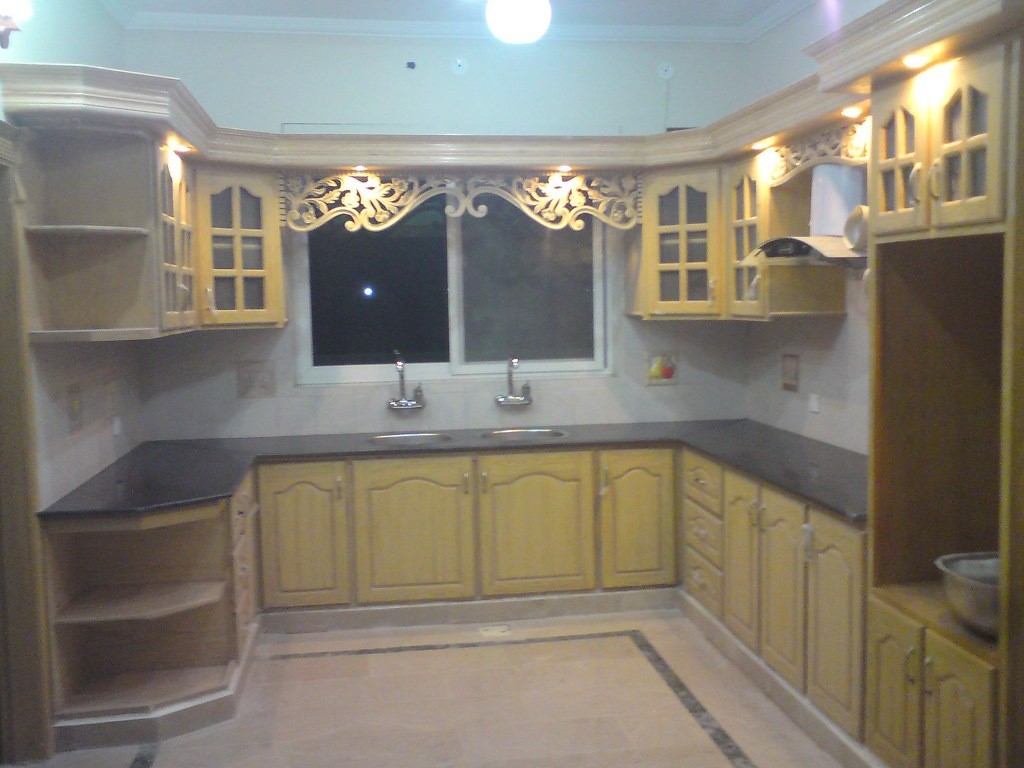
[16,116,286,342]
[596,446,680,592]
[627,115,868,320]
[255,457,356,614]
[800,0,1024,768]
[38,466,256,722]
[345,448,597,607]
[677,445,870,749]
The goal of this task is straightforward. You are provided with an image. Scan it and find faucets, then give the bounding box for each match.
[395,359,405,400]
[507,356,520,395]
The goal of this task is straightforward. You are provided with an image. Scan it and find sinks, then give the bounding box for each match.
[482,428,568,441]
[365,432,455,446]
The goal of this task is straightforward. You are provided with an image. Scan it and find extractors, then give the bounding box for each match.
[740,163,869,266]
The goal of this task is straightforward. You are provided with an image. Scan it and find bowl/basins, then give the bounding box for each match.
[933,550,999,642]
